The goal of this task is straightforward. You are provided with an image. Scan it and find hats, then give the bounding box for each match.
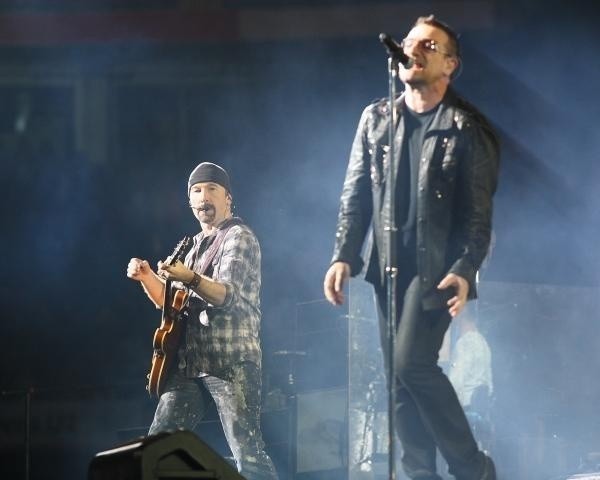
[184,159,234,198]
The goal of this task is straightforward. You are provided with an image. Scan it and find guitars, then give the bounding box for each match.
[147,237,197,398]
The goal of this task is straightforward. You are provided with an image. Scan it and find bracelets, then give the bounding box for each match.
[183,272,202,290]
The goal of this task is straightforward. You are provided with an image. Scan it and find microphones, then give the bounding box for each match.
[188,204,208,212]
[375,29,415,70]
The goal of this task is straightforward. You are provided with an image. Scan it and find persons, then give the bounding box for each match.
[126,162,280,480]
[323,13,498,480]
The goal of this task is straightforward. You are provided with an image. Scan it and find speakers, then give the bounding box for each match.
[87,428,249,480]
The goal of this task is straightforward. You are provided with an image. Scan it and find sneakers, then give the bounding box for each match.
[472,448,496,480]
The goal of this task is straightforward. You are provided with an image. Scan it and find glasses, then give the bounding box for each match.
[400,36,453,60]
